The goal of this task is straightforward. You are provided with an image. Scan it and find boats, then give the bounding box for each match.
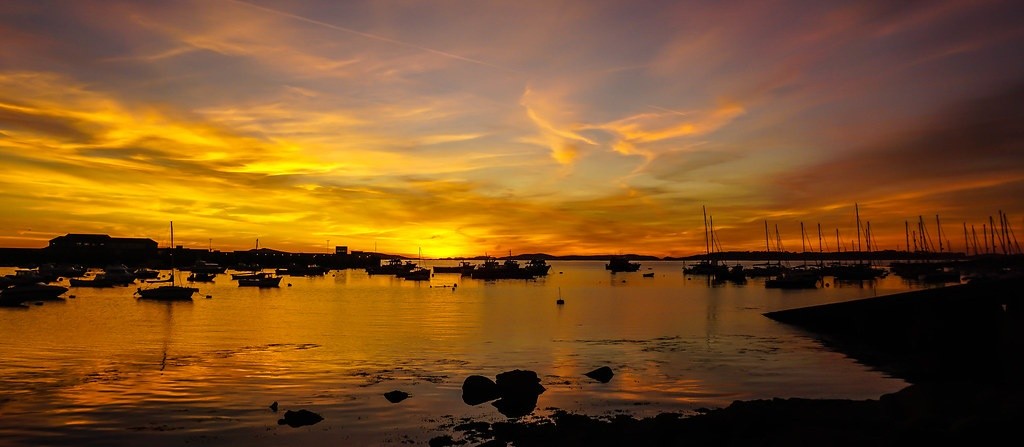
[191,260,229,274]
[364,249,551,280]
[239,273,283,288]
[187,273,216,282]
[231,238,275,279]
[682,204,1024,289]
[606,256,641,273]
[136,220,199,301]
[276,261,329,276]
[0,250,161,300]
